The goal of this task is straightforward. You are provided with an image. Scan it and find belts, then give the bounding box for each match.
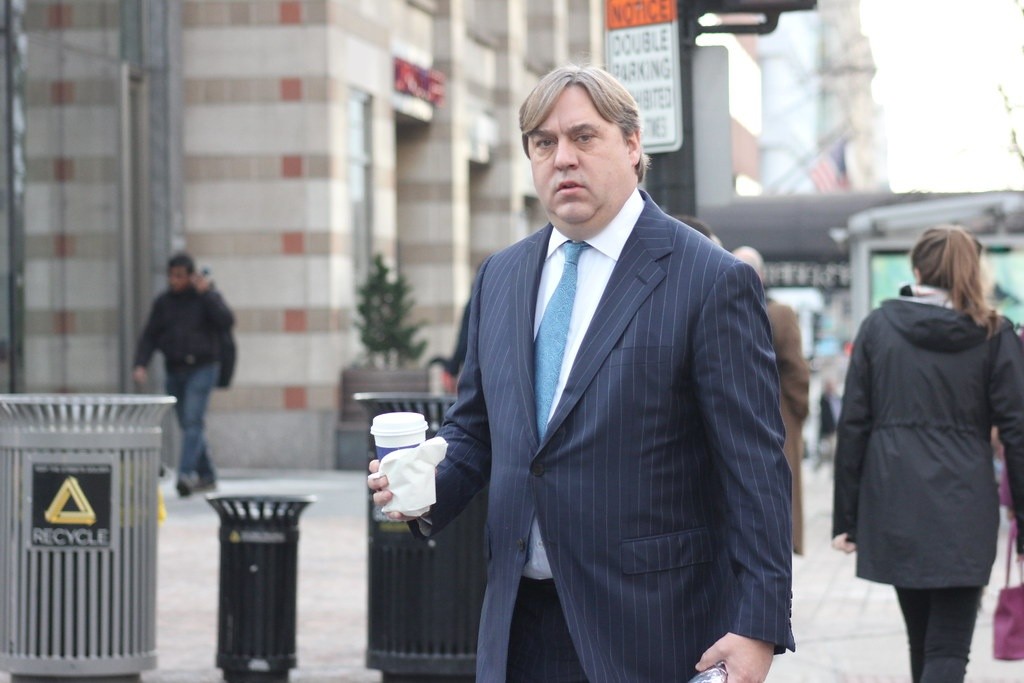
[172,355,215,365]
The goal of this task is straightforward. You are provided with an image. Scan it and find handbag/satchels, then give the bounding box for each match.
[991,520,1023,661]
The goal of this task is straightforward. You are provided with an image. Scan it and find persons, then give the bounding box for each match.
[367,66,796,683]
[664,212,810,558]
[832,224,1024,683]
[132,252,235,495]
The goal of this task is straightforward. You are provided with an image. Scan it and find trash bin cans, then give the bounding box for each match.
[351,389,485,682]
[0,393,179,683]
[206,492,318,683]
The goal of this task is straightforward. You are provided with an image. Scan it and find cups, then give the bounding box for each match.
[370,411,429,462]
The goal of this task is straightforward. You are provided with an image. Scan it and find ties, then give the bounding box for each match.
[531,242,591,449]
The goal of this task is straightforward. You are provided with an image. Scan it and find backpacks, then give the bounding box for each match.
[200,285,237,388]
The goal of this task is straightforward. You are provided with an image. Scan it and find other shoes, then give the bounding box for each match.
[176,475,192,497]
[193,481,216,491]
